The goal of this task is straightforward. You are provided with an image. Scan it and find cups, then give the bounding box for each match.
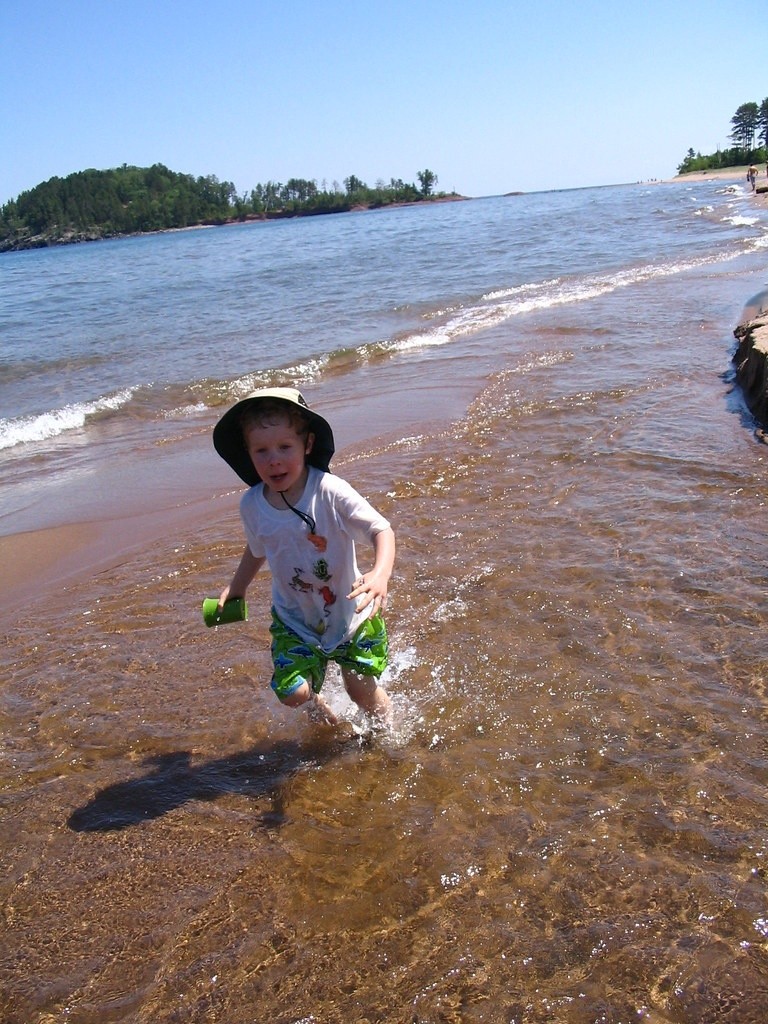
[202,598,247,627]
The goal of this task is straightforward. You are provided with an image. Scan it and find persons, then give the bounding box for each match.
[748,163,758,190]
[213,388,396,715]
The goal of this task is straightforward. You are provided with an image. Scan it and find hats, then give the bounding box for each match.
[213,387,335,534]
